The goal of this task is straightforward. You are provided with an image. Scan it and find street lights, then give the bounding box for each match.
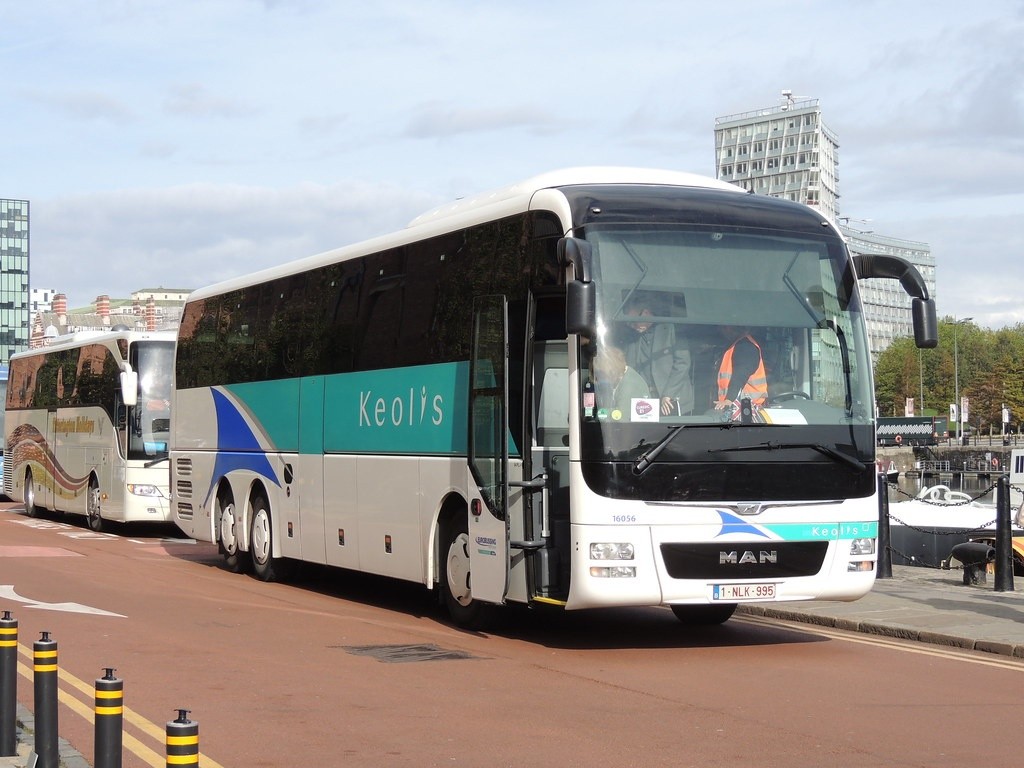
[945,317,973,444]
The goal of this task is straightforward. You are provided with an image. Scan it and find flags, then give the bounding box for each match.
[950,403,956,421]
[1002,406,1011,423]
[963,397,968,422]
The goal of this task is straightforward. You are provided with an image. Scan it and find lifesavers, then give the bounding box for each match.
[895,435,902,442]
[992,458,998,465]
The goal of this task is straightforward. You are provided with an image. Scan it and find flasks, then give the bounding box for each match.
[741,398,752,423]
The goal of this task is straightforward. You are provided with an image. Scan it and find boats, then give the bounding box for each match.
[885,447,1024,580]
[876,459,900,484]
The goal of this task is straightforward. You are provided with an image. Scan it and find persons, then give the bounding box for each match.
[588,346,651,421]
[709,324,768,410]
[565,299,694,418]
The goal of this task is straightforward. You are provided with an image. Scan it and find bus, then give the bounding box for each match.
[0,363,8,501]
[166,165,938,634]
[2,323,178,535]
